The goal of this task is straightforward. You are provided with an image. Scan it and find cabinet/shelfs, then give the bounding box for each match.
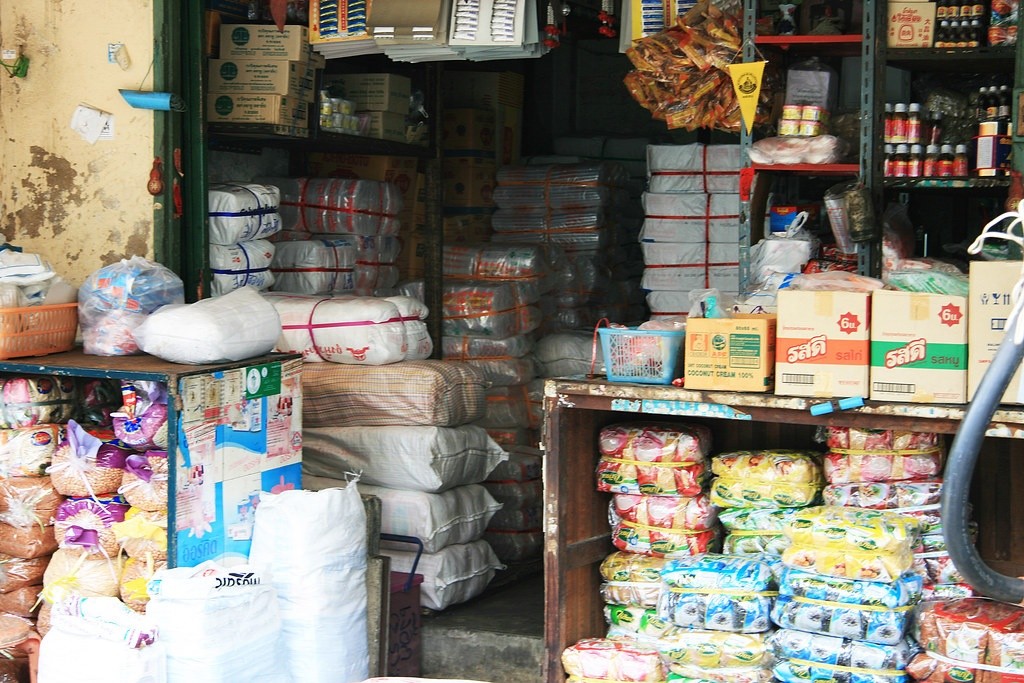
[738,2,1024,306]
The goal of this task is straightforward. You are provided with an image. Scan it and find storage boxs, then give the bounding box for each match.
[205,24,496,285]
[683,254,1024,406]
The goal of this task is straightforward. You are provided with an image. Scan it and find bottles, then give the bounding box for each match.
[978,86,1012,119]
[880,102,969,181]
[932,0,987,48]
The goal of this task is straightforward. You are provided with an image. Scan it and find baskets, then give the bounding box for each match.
[598,327,685,384]
[0,304,78,361]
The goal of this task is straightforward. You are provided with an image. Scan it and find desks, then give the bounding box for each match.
[544,378,1024,683]
[0,346,303,570]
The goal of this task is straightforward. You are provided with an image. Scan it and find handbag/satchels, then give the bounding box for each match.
[77,254,184,357]
